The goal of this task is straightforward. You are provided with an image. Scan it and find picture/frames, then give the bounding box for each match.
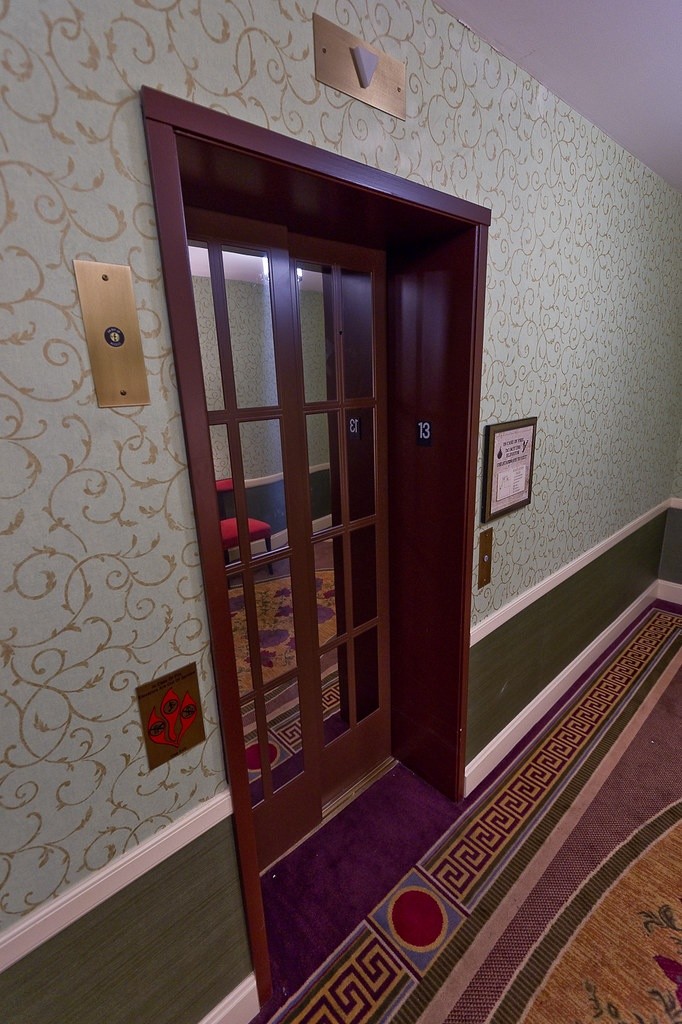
[482,415,539,523]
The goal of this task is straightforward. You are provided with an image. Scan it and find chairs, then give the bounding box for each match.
[216,477,290,586]
